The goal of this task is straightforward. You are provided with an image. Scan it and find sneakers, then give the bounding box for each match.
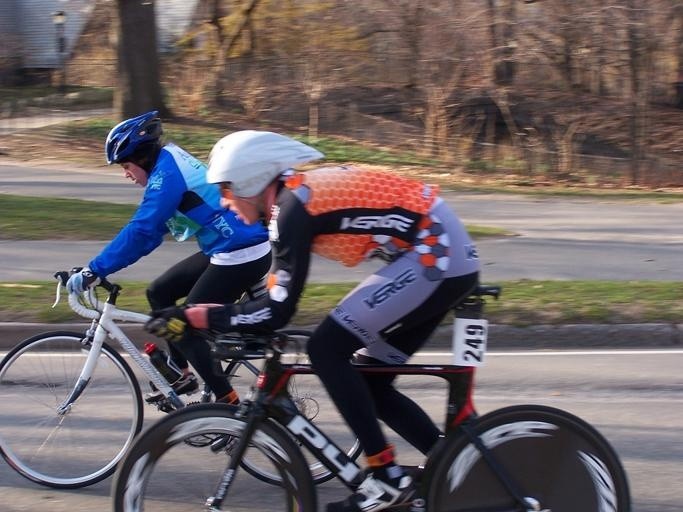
[320,461,414,512]
[142,372,200,403]
[208,386,242,453]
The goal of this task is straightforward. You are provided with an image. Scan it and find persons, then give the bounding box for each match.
[65,109,272,405]
[144,128,481,512]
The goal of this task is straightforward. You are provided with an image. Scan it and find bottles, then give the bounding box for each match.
[142,339,186,388]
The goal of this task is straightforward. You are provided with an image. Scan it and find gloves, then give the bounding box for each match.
[63,265,104,296]
[141,304,193,344]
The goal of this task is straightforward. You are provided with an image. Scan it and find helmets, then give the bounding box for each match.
[103,108,163,166]
[204,128,325,199]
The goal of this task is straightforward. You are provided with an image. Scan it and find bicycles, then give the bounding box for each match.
[109,284,631,512]
[0,267,364,490]
